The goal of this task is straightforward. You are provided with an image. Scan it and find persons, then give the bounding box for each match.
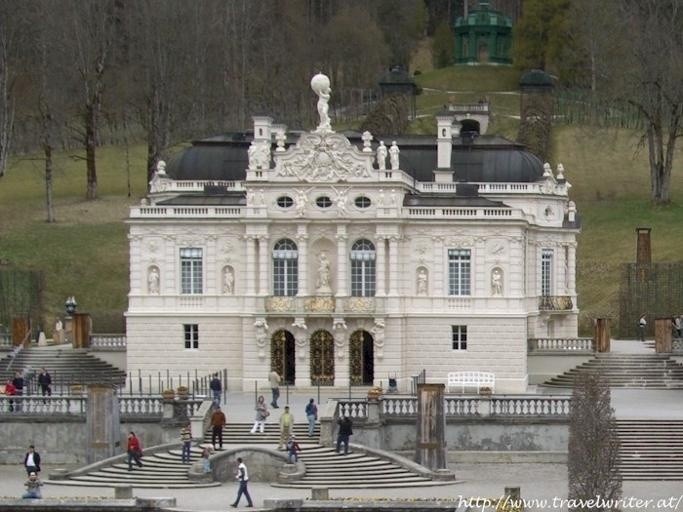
[53,316,62,345]
[304,398,318,438]
[313,85,332,128]
[227,457,253,508]
[4,379,16,412]
[416,270,427,296]
[376,140,387,170]
[491,269,501,297]
[334,414,353,456]
[199,444,216,473]
[247,140,256,169]
[268,367,281,409]
[208,373,222,411]
[313,252,332,289]
[637,312,646,341]
[276,406,294,449]
[248,395,267,434]
[179,425,192,465]
[259,140,270,167]
[147,268,158,295]
[388,139,399,170]
[24,444,41,476]
[222,267,233,295]
[208,406,226,451]
[12,371,24,412]
[37,367,51,405]
[285,433,301,465]
[21,472,44,500]
[125,431,142,472]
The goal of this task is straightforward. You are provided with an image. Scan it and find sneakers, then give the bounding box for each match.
[246,504,252,507]
[230,504,237,507]
